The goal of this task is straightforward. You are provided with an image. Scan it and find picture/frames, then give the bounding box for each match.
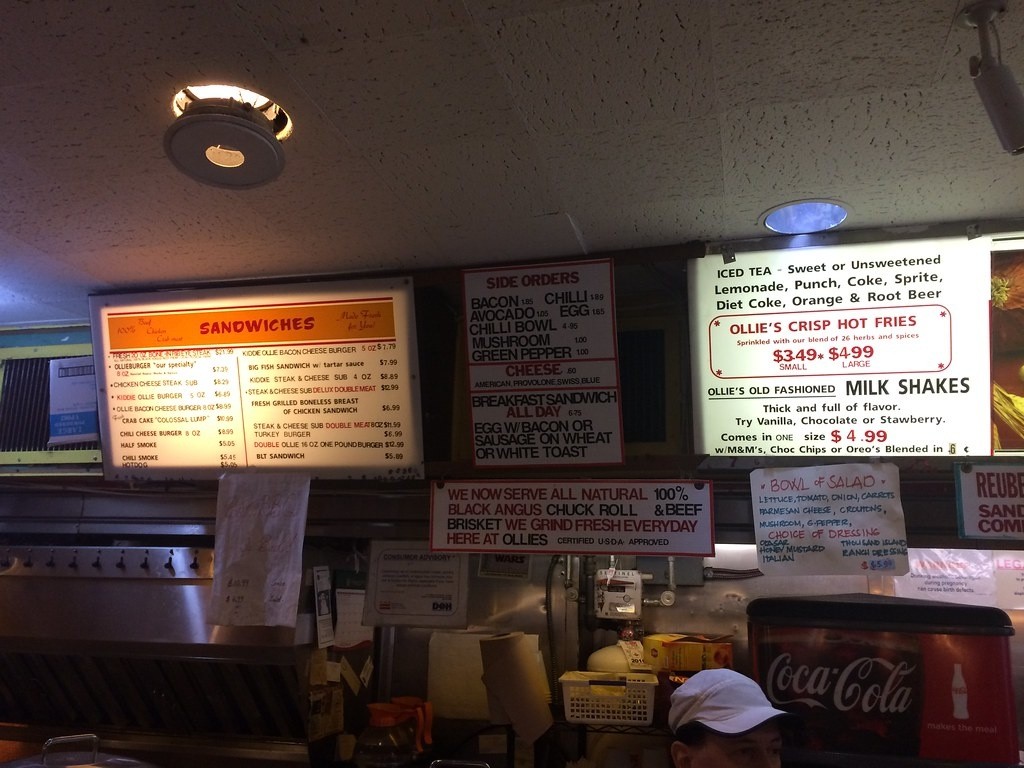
[953,462,1024,540]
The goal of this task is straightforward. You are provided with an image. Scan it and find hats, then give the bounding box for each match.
[669,668,794,736]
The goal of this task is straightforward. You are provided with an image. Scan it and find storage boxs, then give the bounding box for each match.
[426,631,539,721]
[411,714,516,768]
[642,633,734,671]
[745,593,1021,765]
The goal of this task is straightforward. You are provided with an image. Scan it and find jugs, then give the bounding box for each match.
[357,693,434,768]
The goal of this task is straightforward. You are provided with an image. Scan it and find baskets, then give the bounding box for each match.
[558,667,659,725]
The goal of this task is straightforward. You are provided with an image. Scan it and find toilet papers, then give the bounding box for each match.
[479,630,554,746]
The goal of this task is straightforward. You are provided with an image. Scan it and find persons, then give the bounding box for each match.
[668,669,790,768]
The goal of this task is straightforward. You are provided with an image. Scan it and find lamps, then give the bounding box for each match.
[162,84,293,190]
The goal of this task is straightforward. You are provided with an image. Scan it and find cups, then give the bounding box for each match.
[338,735,355,760]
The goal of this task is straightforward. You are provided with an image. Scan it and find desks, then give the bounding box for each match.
[549,702,675,737]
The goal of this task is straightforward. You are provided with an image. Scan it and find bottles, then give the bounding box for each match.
[951,662,969,719]
